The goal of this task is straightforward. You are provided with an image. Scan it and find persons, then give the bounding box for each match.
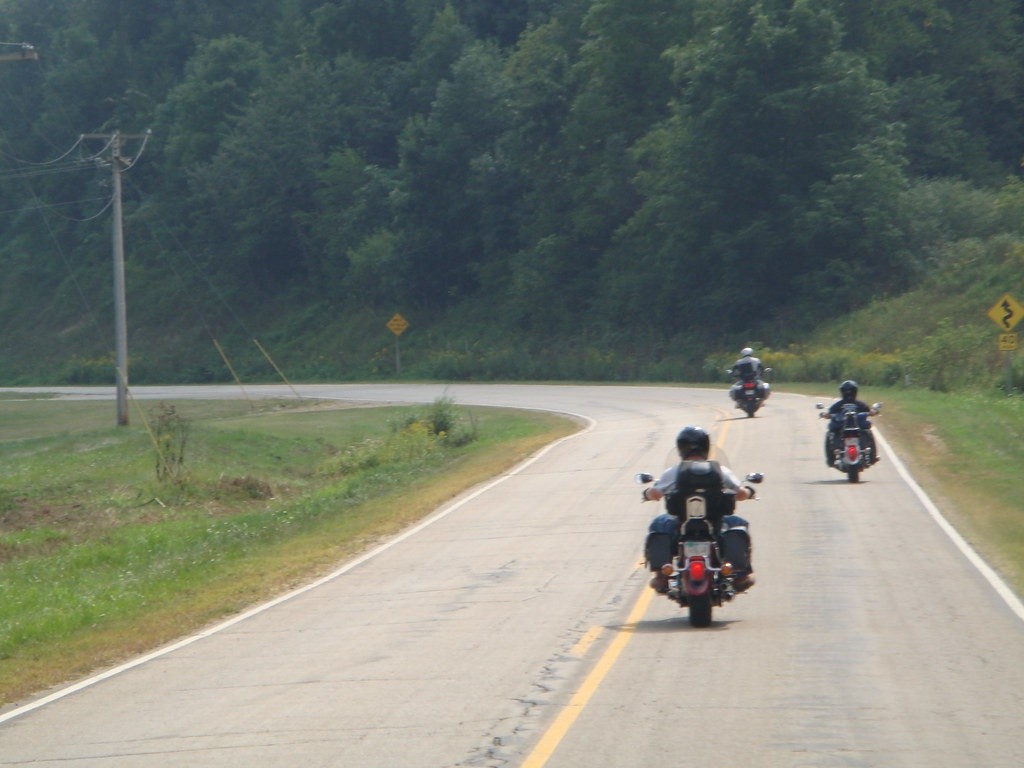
[822,380,879,463]
[644,427,756,589]
[728,348,771,401]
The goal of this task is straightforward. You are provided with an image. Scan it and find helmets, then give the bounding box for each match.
[741,347,753,357]
[839,380,858,402]
[676,426,710,457]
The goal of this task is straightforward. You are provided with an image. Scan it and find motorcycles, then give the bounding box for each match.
[725,367,773,418]
[816,402,882,483]
[634,471,764,628]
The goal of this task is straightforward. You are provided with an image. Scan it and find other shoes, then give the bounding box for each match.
[735,574,755,588]
[649,577,667,589]
